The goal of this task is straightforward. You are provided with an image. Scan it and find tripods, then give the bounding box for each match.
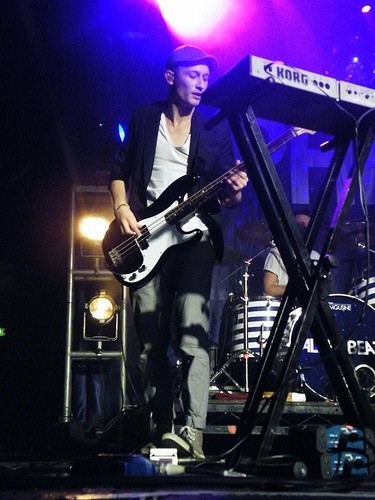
[208,245,274,393]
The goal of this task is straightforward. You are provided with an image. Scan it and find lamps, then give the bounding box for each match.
[79,213,112,257]
[84,290,118,340]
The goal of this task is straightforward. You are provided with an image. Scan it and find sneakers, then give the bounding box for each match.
[161,425,205,461]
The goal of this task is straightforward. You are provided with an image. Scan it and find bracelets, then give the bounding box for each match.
[113,203,129,215]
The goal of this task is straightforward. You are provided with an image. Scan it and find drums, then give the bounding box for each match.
[275,293,375,403]
[351,269,375,309]
[225,291,283,385]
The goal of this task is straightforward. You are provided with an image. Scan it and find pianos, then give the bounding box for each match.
[201,54,375,479]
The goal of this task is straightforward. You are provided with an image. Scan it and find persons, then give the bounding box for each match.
[264,212,311,293]
[107,45,249,460]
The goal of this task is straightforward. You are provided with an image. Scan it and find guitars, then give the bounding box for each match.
[103,126,316,290]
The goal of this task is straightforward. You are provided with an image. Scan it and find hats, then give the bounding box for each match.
[166,44,219,73]
